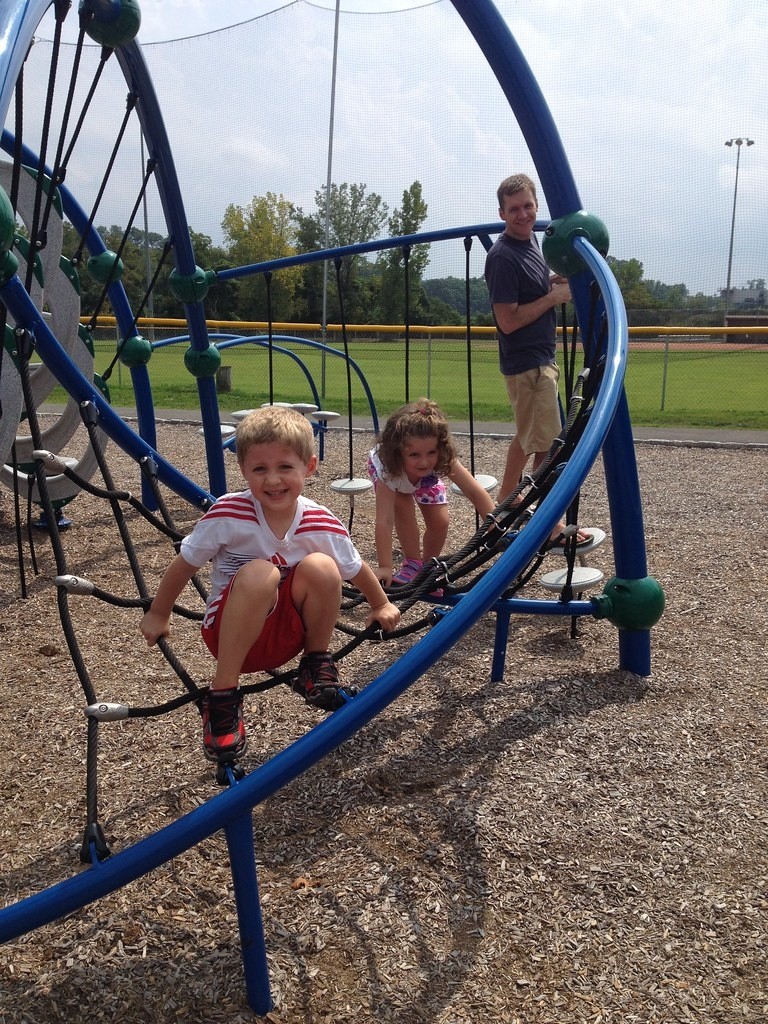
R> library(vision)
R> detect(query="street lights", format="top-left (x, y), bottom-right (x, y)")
top-left (724, 137), bottom-right (755, 315)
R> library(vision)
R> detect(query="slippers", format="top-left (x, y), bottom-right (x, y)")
top-left (494, 502), bottom-right (535, 516)
top-left (548, 535), bottom-right (594, 548)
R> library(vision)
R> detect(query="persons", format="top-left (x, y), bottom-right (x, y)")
top-left (483, 174), bottom-right (597, 550)
top-left (369, 397), bottom-right (500, 601)
top-left (137, 405), bottom-right (403, 763)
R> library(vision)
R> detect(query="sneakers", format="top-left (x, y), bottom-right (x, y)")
top-left (293, 651), bottom-right (358, 711)
top-left (203, 681), bottom-right (248, 762)
top-left (423, 561), bottom-right (443, 597)
top-left (389, 559), bottom-right (423, 587)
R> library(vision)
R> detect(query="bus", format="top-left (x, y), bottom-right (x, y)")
top-left (659, 335), bottom-right (710, 343)
